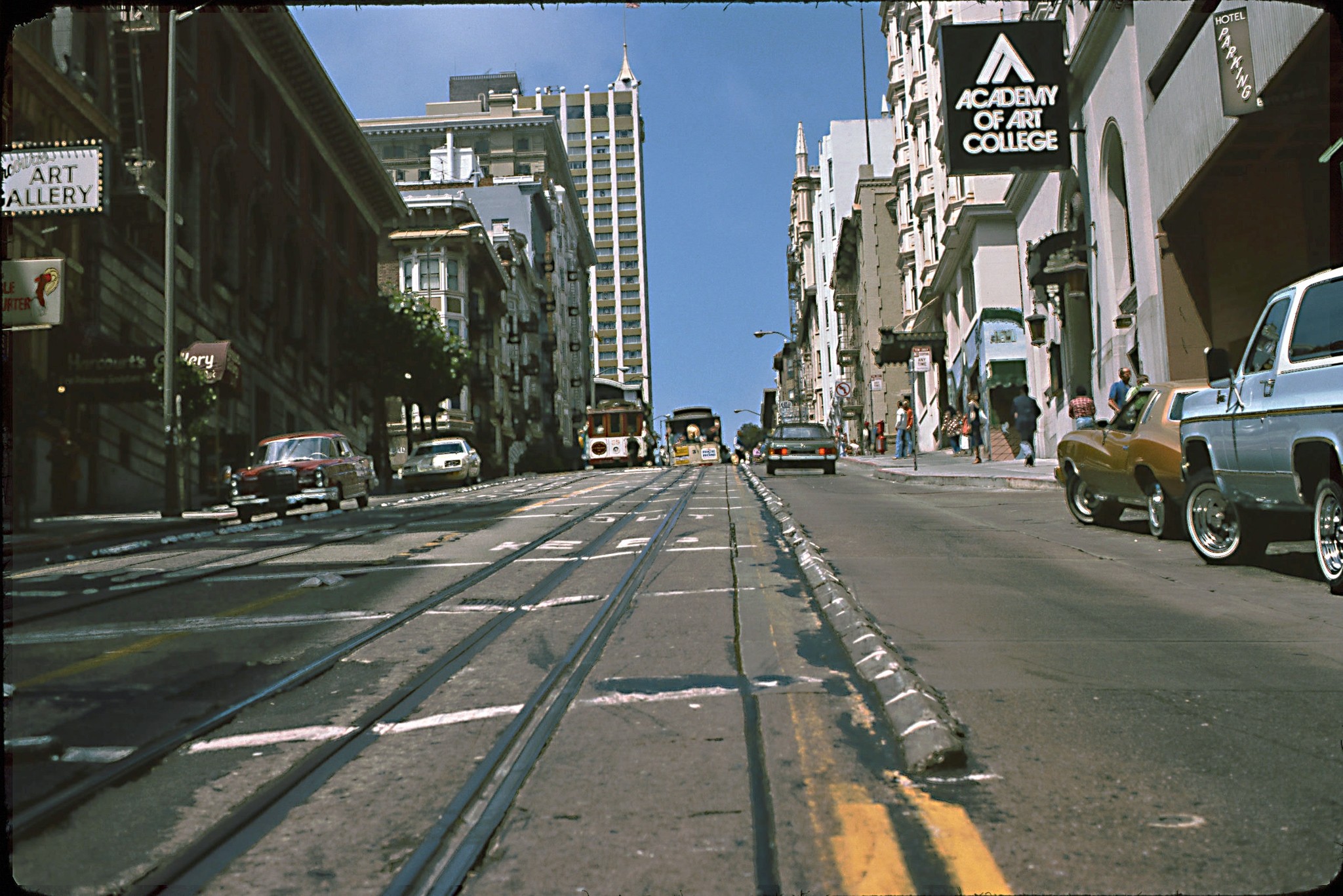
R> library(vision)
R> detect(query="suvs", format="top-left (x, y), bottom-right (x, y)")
top-left (1177, 264), bottom-right (1343, 595)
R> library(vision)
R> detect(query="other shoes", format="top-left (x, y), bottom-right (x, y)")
top-left (972, 457), bottom-right (981, 463)
top-left (1025, 453), bottom-right (1035, 467)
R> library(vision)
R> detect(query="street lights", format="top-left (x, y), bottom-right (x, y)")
top-left (752, 329), bottom-right (803, 424)
top-left (423, 219), bottom-right (483, 445)
top-left (590, 366), bottom-right (630, 410)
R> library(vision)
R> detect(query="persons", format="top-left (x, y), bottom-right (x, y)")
top-left (892, 400), bottom-right (913, 460)
top-left (1013, 384), bottom-right (1041, 467)
top-left (1069, 385), bottom-right (1095, 431)
top-left (733, 430), bottom-right (770, 465)
top-left (835, 421), bottom-right (870, 457)
top-left (627, 432), bottom-right (640, 467)
top-left (966, 392), bottom-right (984, 464)
top-left (1108, 368), bottom-right (1150, 414)
top-left (941, 405), bottom-right (966, 457)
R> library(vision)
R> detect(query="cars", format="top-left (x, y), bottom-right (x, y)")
top-left (1051, 379), bottom-right (1221, 542)
top-left (763, 422), bottom-right (839, 475)
top-left (228, 429), bottom-right (377, 524)
top-left (401, 437), bottom-right (483, 493)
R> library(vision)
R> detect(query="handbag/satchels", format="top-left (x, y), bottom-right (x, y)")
top-left (979, 409), bottom-right (987, 424)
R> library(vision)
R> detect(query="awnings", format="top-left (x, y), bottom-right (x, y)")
top-left (56, 342), bottom-right (243, 403)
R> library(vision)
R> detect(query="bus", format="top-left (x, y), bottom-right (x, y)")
top-left (665, 405), bottom-right (725, 466)
top-left (584, 397), bottom-right (659, 469)
